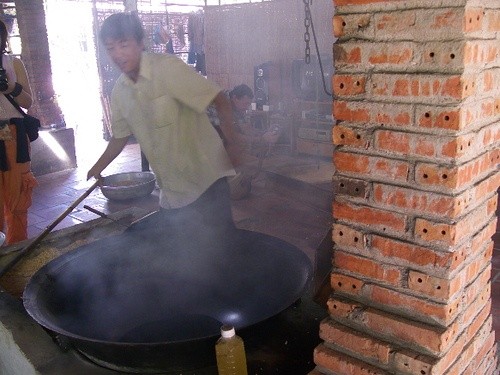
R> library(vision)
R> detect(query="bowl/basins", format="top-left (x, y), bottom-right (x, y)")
top-left (98, 171), bottom-right (156, 201)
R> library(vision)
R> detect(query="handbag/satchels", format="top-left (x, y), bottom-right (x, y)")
top-left (25, 115), bottom-right (41, 142)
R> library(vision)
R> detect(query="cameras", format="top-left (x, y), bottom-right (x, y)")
top-left (0, 66), bottom-right (8, 91)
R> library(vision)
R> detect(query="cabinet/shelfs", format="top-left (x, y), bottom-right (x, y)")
top-left (298, 98), bottom-right (335, 124)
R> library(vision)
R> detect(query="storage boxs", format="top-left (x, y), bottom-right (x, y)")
top-left (295, 128), bottom-right (334, 158)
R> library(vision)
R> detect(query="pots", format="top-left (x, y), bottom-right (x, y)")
top-left (27, 227), bottom-right (312, 368)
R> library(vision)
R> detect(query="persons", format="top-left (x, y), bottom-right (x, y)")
top-left (87, 12), bottom-right (284, 226)
top-left (0, 20), bottom-right (38, 248)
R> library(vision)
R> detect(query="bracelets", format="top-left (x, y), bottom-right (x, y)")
top-left (11, 83), bottom-right (23, 96)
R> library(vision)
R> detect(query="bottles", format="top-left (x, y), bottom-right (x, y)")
top-left (215, 324), bottom-right (248, 375)
